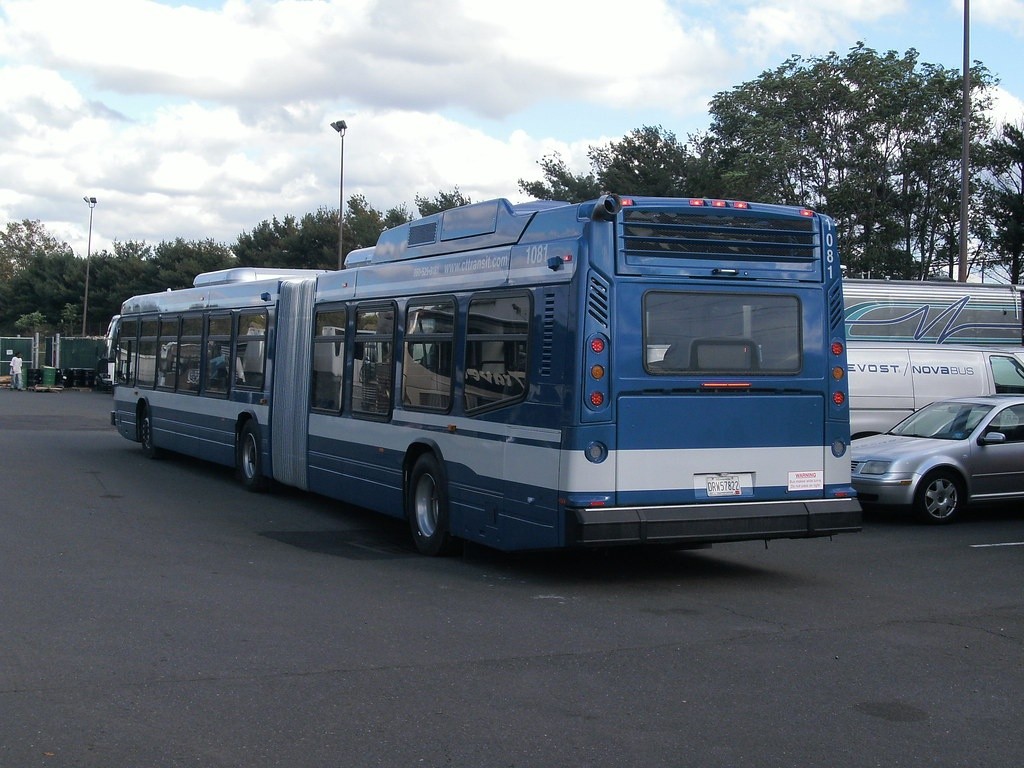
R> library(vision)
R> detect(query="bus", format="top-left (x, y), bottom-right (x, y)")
top-left (94, 192), bottom-right (865, 560)
top-left (741, 277), bottom-right (1024, 441)
top-left (376, 306), bottom-right (530, 412)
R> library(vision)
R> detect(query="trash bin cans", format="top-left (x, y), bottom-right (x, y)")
top-left (27, 368), bottom-right (43, 386)
top-left (14, 367), bottom-right (27, 387)
top-left (56, 368), bottom-right (94, 387)
top-left (40, 365), bottom-right (55, 385)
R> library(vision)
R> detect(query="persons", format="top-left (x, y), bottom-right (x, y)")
top-left (9, 352), bottom-right (23, 392)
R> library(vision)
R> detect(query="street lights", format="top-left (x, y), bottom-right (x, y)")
top-left (81, 195), bottom-right (98, 337)
top-left (329, 119), bottom-right (348, 271)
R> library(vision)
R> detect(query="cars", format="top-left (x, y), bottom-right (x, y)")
top-left (851, 393), bottom-right (1024, 526)
